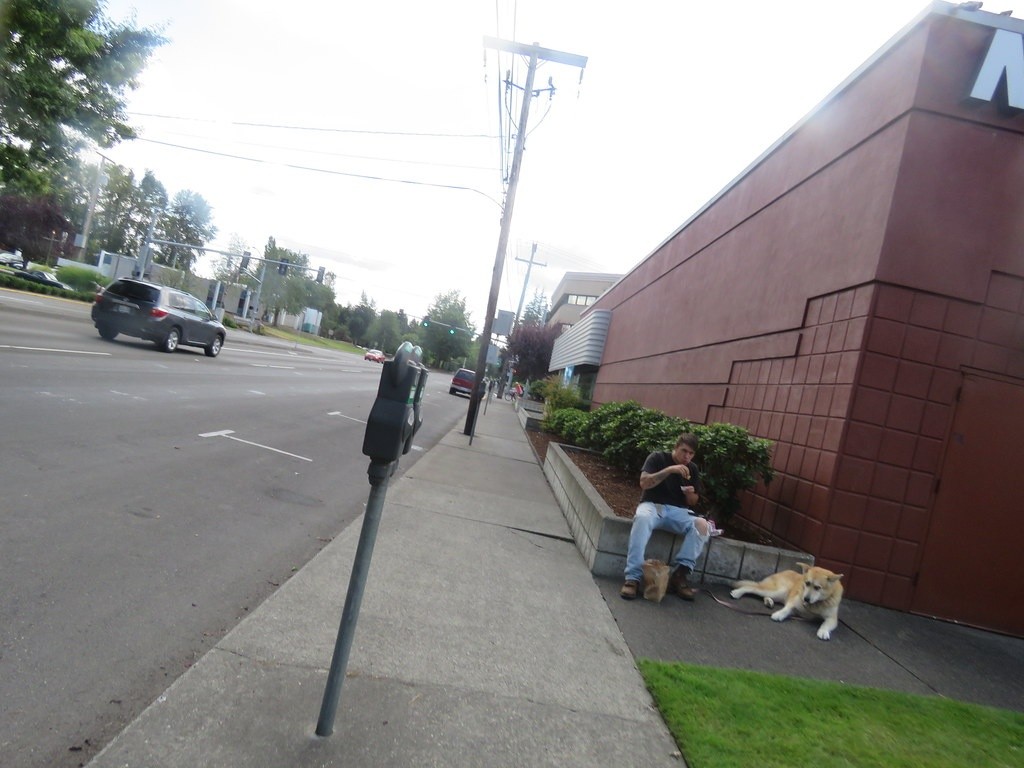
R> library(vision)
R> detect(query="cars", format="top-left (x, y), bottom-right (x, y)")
top-left (0, 253), bottom-right (23, 270)
top-left (13, 268), bottom-right (74, 290)
top-left (363, 348), bottom-right (385, 365)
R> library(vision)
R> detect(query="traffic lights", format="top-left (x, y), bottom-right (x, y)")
top-left (397, 309), bottom-right (403, 319)
top-left (422, 315), bottom-right (430, 327)
top-left (446, 318), bottom-right (457, 335)
top-left (240, 265), bottom-right (245, 275)
top-left (227, 255), bottom-right (234, 267)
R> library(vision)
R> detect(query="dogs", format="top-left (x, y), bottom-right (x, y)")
top-left (730, 562), bottom-right (845, 640)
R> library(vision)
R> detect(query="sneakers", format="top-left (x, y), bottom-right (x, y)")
top-left (620, 579), bottom-right (639, 598)
top-left (669, 574), bottom-right (695, 601)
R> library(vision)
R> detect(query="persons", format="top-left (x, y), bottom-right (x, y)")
top-left (620, 433), bottom-right (710, 601)
top-left (515, 382), bottom-right (525, 398)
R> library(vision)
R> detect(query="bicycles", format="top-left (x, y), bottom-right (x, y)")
top-left (504, 388), bottom-right (518, 401)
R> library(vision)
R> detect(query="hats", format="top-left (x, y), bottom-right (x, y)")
top-left (515, 382), bottom-right (518, 385)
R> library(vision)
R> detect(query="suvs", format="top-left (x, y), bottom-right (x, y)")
top-left (89, 276), bottom-right (227, 358)
top-left (449, 368), bottom-right (486, 400)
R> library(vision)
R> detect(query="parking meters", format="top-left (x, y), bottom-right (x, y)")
top-left (484, 379), bottom-right (494, 415)
top-left (309, 341), bottom-right (427, 738)
top-left (469, 381), bottom-right (487, 445)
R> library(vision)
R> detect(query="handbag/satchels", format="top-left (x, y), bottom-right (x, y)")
top-left (642, 558), bottom-right (670, 603)
